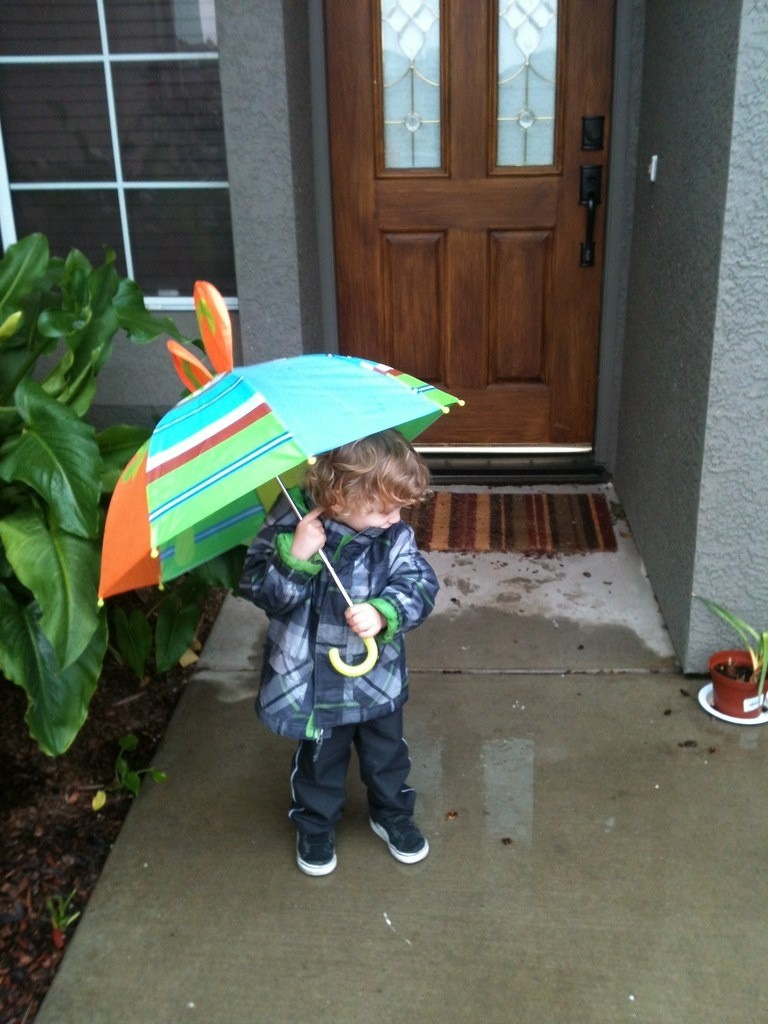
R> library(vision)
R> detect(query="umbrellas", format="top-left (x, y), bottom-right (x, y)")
top-left (97, 282), bottom-right (465, 678)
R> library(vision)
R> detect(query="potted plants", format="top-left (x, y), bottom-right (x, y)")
top-left (691, 595), bottom-right (768, 725)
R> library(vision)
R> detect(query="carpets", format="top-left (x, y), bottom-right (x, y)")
top-left (400, 493), bottom-right (618, 552)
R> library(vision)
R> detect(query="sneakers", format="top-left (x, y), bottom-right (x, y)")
top-left (295, 818), bottom-right (337, 878)
top-left (369, 812), bottom-right (429, 863)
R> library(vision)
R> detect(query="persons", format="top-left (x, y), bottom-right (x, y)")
top-left (239, 429), bottom-right (439, 876)
